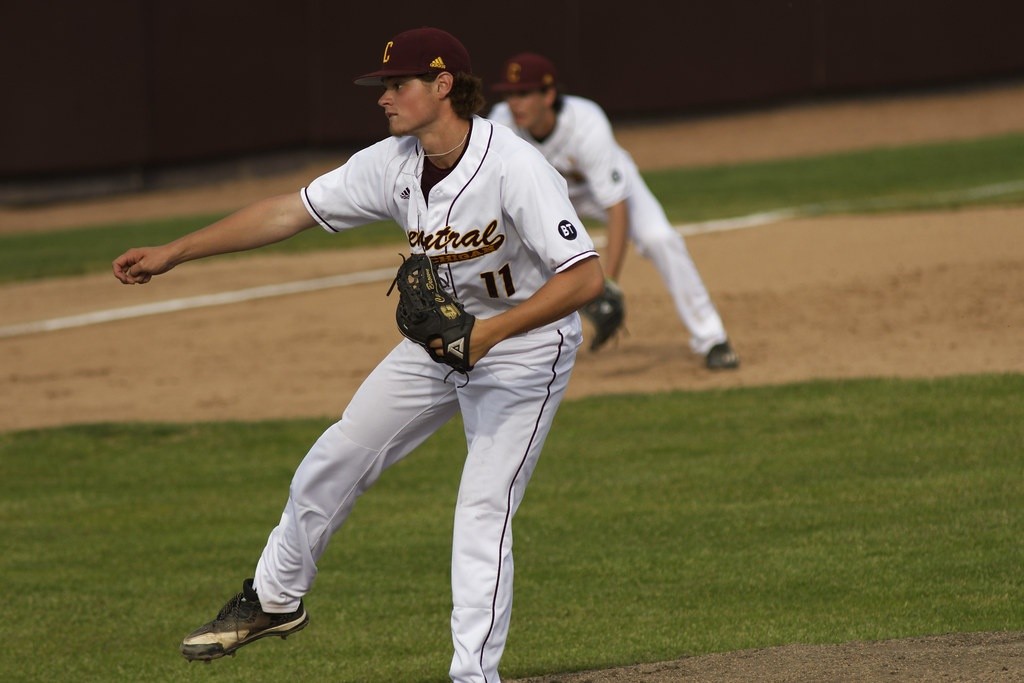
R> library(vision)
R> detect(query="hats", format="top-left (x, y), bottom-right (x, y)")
top-left (491, 54), bottom-right (554, 91)
top-left (351, 27), bottom-right (471, 86)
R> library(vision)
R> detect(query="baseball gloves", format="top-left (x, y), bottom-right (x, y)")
top-left (384, 251), bottom-right (476, 390)
top-left (581, 278), bottom-right (632, 353)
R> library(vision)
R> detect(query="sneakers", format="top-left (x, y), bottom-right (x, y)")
top-left (179, 578), bottom-right (309, 664)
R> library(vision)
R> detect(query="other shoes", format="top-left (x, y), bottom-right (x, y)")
top-left (705, 343), bottom-right (739, 368)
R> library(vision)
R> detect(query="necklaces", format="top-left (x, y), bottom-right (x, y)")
top-left (421, 128), bottom-right (470, 157)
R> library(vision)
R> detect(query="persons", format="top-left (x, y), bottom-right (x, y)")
top-left (111, 26), bottom-right (605, 683)
top-left (486, 52), bottom-right (740, 370)
